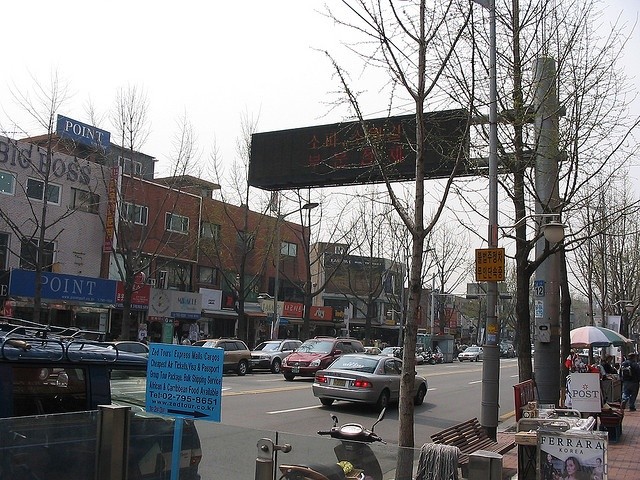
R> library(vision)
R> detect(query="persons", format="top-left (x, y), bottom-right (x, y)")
top-left (543, 454), bottom-right (558, 480)
top-left (590, 458), bottom-right (603, 480)
top-left (182, 333), bottom-right (191, 345)
top-left (561, 457), bottom-right (581, 480)
top-left (139, 337), bottom-right (148, 346)
top-left (601, 355), bottom-right (617, 374)
top-left (618, 352), bottom-right (640, 411)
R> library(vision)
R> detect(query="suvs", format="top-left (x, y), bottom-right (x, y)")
top-left (250, 337), bottom-right (304, 374)
top-left (281, 336), bottom-right (364, 380)
top-left (191, 337), bottom-right (251, 376)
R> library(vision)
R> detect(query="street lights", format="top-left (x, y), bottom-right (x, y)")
top-left (601, 300), bottom-right (634, 364)
top-left (386, 310), bottom-right (403, 346)
top-left (274, 203), bottom-right (319, 339)
top-left (481, 214), bottom-right (568, 443)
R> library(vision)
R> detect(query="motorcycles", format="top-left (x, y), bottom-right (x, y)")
top-left (421, 348), bottom-right (444, 365)
top-left (279, 407), bottom-right (387, 480)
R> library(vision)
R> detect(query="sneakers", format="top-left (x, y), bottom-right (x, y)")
top-left (621, 400), bottom-right (627, 409)
top-left (629, 407), bottom-right (636, 411)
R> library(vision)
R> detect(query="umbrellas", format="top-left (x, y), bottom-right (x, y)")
top-left (560, 325), bottom-right (628, 366)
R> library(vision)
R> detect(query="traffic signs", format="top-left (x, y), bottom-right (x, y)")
top-left (146, 342), bottom-right (224, 421)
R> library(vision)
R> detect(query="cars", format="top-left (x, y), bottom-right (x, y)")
top-left (378, 347), bottom-right (404, 357)
top-left (458, 345), bottom-right (483, 362)
top-left (312, 353), bottom-right (428, 412)
top-left (500, 343), bottom-right (515, 358)
top-left (107, 341), bottom-right (149, 371)
top-left (364, 347), bottom-right (382, 355)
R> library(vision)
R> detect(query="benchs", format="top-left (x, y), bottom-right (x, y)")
top-left (431, 417), bottom-right (517, 477)
